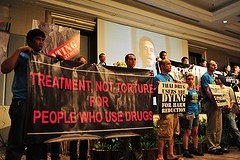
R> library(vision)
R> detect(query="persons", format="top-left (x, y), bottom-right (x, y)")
top-left (216, 76), bottom-right (240, 142)
top-left (37, 21), bottom-right (54, 54)
top-left (117, 53), bottom-right (154, 160)
top-left (1, 28), bottom-right (64, 160)
top-left (69, 56), bottom-right (88, 160)
top-left (154, 59), bottom-right (184, 160)
top-left (98, 53), bottom-right (107, 66)
top-left (180, 74), bottom-right (204, 158)
top-left (232, 65), bottom-right (240, 75)
top-left (138, 36), bottom-right (156, 70)
top-left (223, 65), bottom-right (230, 73)
top-left (155, 50), bottom-right (167, 112)
top-left (201, 59), bottom-right (229, 155)
top-left (182, 57), bottom-right (189, 64)
top-left (198, 59), bottom-right (207, 67)
top-left (49, 25), bottom-right (63, 49)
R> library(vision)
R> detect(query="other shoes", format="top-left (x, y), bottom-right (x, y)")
top-left (183, 152), bottom-right (193, 158)
top-left (168, 155), bottom-right (184, 160)
top-left (192, 148), bottom-right (204, 156)
top-left (218, 149), bottom-right (228, 152)
top-left (208, 150), bottom-right (223, 155)
